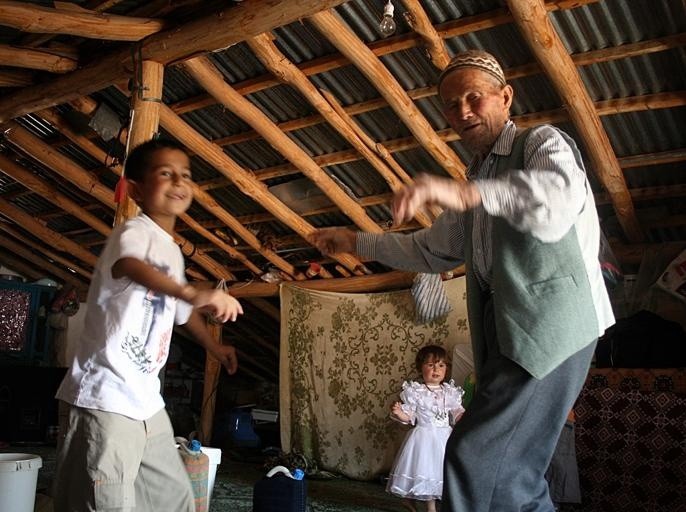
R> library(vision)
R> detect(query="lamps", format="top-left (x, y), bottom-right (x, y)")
top-left (379, 0), bottom-right (396, 36)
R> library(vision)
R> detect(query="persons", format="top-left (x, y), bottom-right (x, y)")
top-left (56, 138), bottom-right (244, 512)
top-left (305, 51), bottom-right (616, 511)
top-left (386, 346), bottom-right (465, 511)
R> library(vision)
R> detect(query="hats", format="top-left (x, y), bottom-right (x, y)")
top-left (437, 50), bottom-right (507, 85)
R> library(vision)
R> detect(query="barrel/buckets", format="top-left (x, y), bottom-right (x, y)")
top-left (0, 453), bottom-right (42, 512)
top-left (195, 447), bottom-right (221, 511)
top-left (178, 440), bottom-right (208, 512)
top-left (253, 465), bottom-right (306, 512)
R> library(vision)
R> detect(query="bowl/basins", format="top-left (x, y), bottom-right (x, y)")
top-left (0, 274), bottom-right (23, 283)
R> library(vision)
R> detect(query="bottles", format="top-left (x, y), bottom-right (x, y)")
top-left (624, 274), bottom-right (639, 304)
